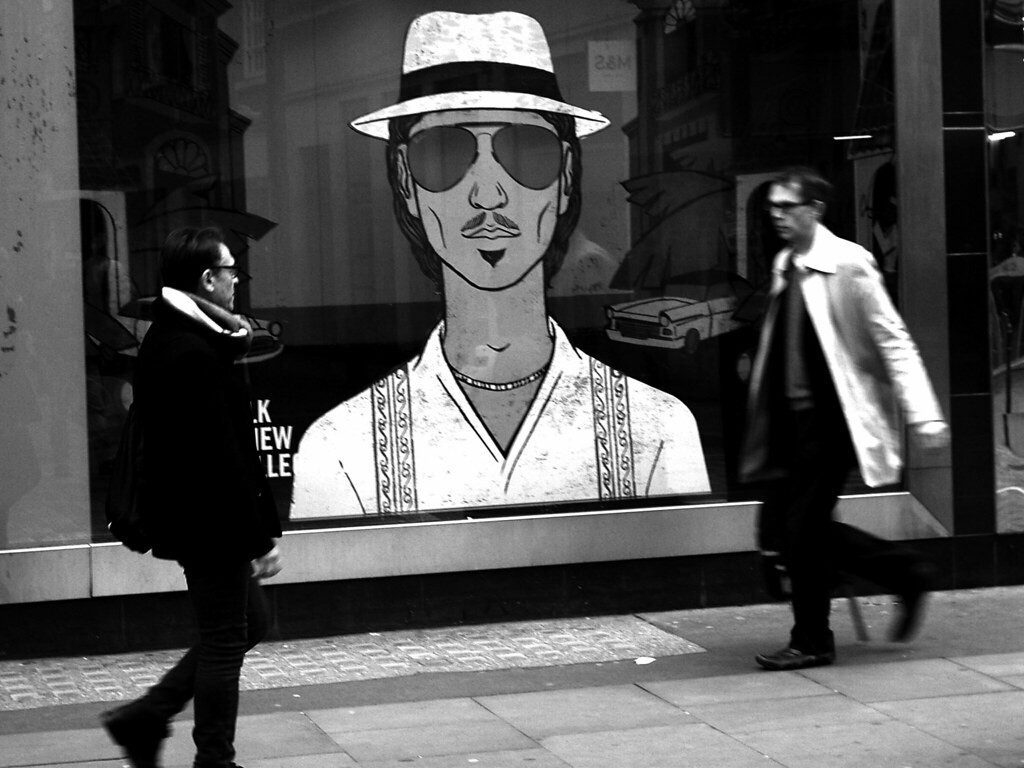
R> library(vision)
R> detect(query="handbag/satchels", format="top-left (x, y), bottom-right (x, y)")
top-left (107, 405), bottom-right (156, 552)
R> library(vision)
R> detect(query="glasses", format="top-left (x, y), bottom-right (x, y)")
top-left (768, 199), bottom-right (813, 211)
top-left (215, 261), bottom-right (246, 282)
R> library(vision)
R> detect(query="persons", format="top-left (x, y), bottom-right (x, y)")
top-left (98, 225), bottom-right (284, 768)
top-left (747, 172), bottom-right (951, 670)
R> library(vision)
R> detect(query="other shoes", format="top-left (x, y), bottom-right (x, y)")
top-left (100, 704), bottom-right (171, 766)
top-left (194, 755), bottom-right (240, 768)
top-left (754, 632), bottom-right (834, 669)
top-left (885, 567), bottom-right (924, 640)
